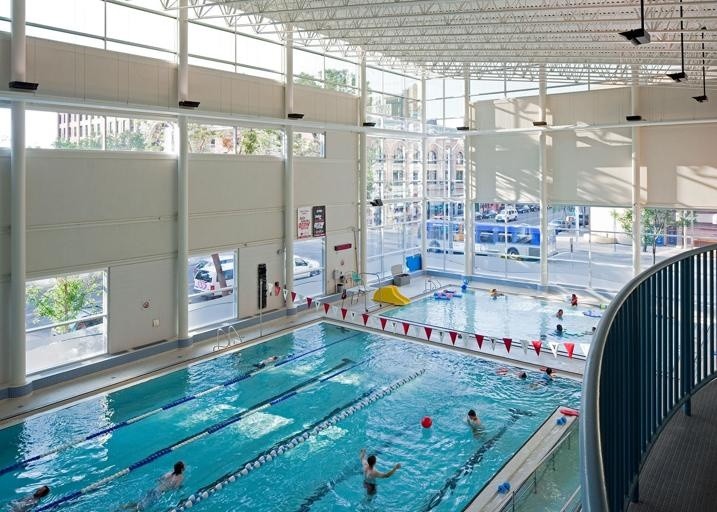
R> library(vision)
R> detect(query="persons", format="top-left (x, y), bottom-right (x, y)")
top-left (513, 371), bottom-right (527, 380)
top-left (250, 355), bottom-right (277, 371)
top-left (116, 460), bottom-right (185, 511)
top-left (464, 409), bottom-right (487, 442)
top-left (570, 293), bottom-right (578, 307)
top-left (547, 324), bottom-right (563, 337)
top-left (6, 484), bottom-right (50, 512)
top-left (549, 308), bottom-right (564, 321)
top-left (528, 367), bottom-right (552, 390)
top-left (488, 288), bottom-right (503, 296)
top-left (359, 448), bottom-right (401, 503)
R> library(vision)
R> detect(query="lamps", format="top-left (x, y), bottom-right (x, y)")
top-left (533, 121), bottom-right (548, 127)
top-left (626, 115), bottom-right (642, 121)
top-left (617, 1), bottom-right (710, 103)
top-left (8, 81), bottom-right (39, 91)
top-left (288, 112), bottom-right (305, 118)
top-left (362, 122), bottom-right (376, 128)
top-left (456, 126), bottom-right (470, 131)
top-left (179, 99), bottom-right (201, 108)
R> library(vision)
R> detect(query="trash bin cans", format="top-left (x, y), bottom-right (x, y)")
top-left (337, 284), bottom-right (343, 294)
top-left (394, 274), bottom-right (410, 287)
top-left (406, 255), bottom-right (421, 273)
top-left (668, 226), bottom-right (676, 244)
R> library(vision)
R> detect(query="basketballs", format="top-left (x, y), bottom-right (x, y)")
top-left (421, 416), bottom-right (433, 428)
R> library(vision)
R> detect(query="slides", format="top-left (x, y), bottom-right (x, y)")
top-left (390, 286), bottom-right (411, 305)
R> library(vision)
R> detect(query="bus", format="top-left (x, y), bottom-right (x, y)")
top-left (416, 218), bottom-right (558, 262)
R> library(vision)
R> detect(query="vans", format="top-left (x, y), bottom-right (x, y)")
top-left (496, 207), bottom-right (519, 221)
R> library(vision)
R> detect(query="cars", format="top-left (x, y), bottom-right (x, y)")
top-left (461, 209), bottom-right (497, 220)
top-left (192, 250), bottom-right (320, 296)
top-left (518, 205), bottom-right (540, 213)
top-left (549, 214), bottom-right (589, 228)
